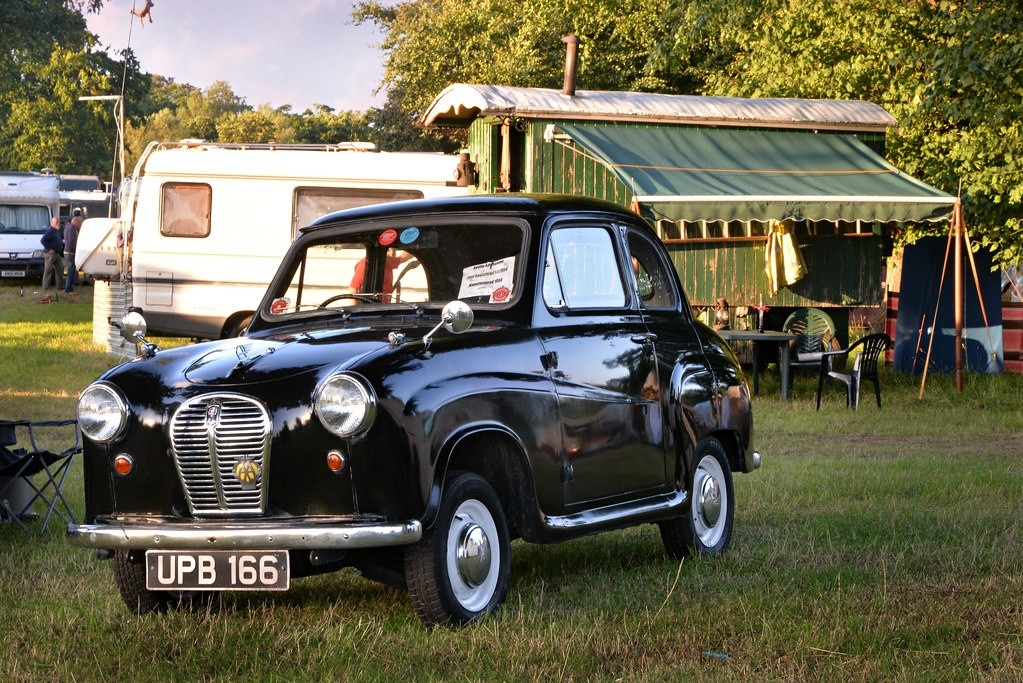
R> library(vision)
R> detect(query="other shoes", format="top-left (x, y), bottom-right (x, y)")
top-left (68, 292), bottom-right (79, 294)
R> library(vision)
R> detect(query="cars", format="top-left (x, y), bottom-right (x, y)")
top-left (65, 190), bottom-right (761, 628)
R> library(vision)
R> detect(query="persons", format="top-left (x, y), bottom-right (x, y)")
top-left (350, 247), bottom-right (414, 306)
top-left (41, 216), bottom-right (84, 295)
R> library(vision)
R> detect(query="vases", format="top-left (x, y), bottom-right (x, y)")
top-left (758, 315), bottom-right (764, 333)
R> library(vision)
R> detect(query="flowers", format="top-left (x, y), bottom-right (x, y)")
top-left (752, 302), bottom-right (770, 326)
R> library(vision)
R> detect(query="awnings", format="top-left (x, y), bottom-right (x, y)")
top-left (554, 122), bottom-right (961, 223)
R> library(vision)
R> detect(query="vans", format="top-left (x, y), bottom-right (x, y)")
top-left (0, 139), bottom-right (477, 341)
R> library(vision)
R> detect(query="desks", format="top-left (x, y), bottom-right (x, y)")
top-left (717, 331), bottom-right (797, 400)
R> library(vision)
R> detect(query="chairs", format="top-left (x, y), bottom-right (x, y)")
top-left (780, 308), bottom-right (836, 389)
top-left (0, 417), bottom-right (84, 536)
top-left (815, 332), bottom-right (891, 413)
